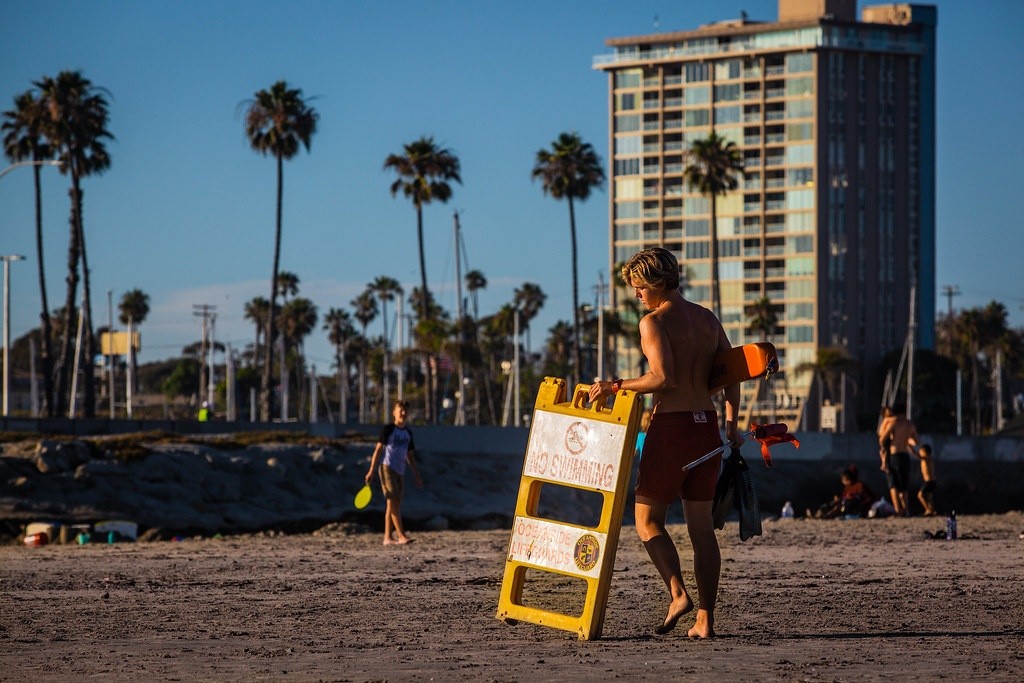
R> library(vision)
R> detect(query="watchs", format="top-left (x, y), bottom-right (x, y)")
top-left (612, 379), bottom-right (624, 392)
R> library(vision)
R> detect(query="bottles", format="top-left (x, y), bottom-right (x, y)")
top-left (946, 514), bottom-right (956, 540)
top-left (782, 501), bottom-right (794, 520)
top-left (23, 532), bottom-right (48, 546)
top-left (78, 531), bottom-right (114, 545)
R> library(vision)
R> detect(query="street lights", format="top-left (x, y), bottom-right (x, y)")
top-left (0, 253), bottom-right (27, 418)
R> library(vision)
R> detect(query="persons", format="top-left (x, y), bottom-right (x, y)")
top-left (589, 247), bottom-right (746, 638)
top-left (878, 400), bottom-right (919, 519)
top-left (198, 400), bottom-right (216, 422)
top-left (633, 409), bottom-right (652, 459)
top-left (834, 464), bottom-right (871, 519)
top-left (365, 400), bottom-right (423, 544)
top-left (907, 442), bottom-right (938, 516)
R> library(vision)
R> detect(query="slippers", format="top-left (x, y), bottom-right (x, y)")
top-left (912, 529), bottom-right (947, 539)
